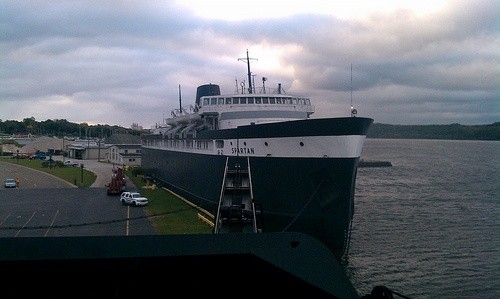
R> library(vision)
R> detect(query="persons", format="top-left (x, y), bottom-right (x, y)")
top-left (15, 177), bottom-right (20, 187)
top-left (74, 176), bottom-right (77, 185)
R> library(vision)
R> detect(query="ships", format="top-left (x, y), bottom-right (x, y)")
top-left (140, 48), bottom-right (374, 266)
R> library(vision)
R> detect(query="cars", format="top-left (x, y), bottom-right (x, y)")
top-left (4, 179), bottom-right (17, 188)
top-left (13, 154), bottom-right (47, 160)
top-left (64, 160), bottom-right (78, 167)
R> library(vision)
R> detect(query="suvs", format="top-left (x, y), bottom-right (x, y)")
top-left (119, 192), bottom-right (149, 207)
